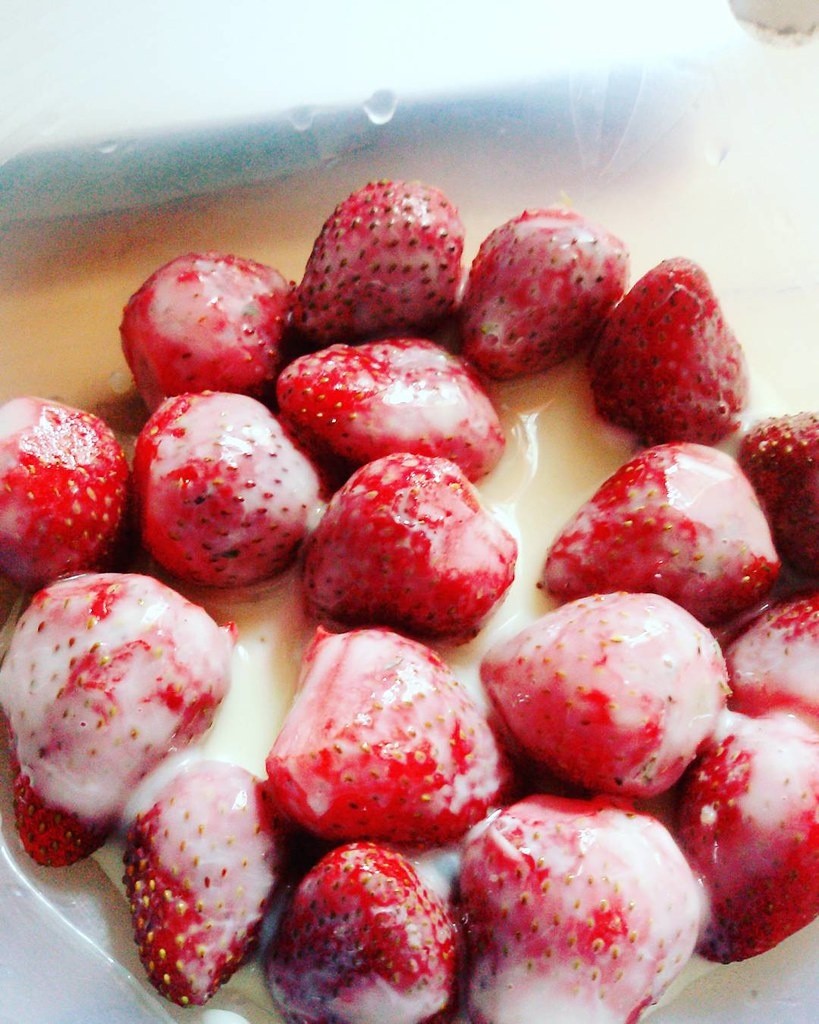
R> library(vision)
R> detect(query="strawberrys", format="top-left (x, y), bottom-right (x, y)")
top-left (0, 179), bottom-right (819, 1023)
